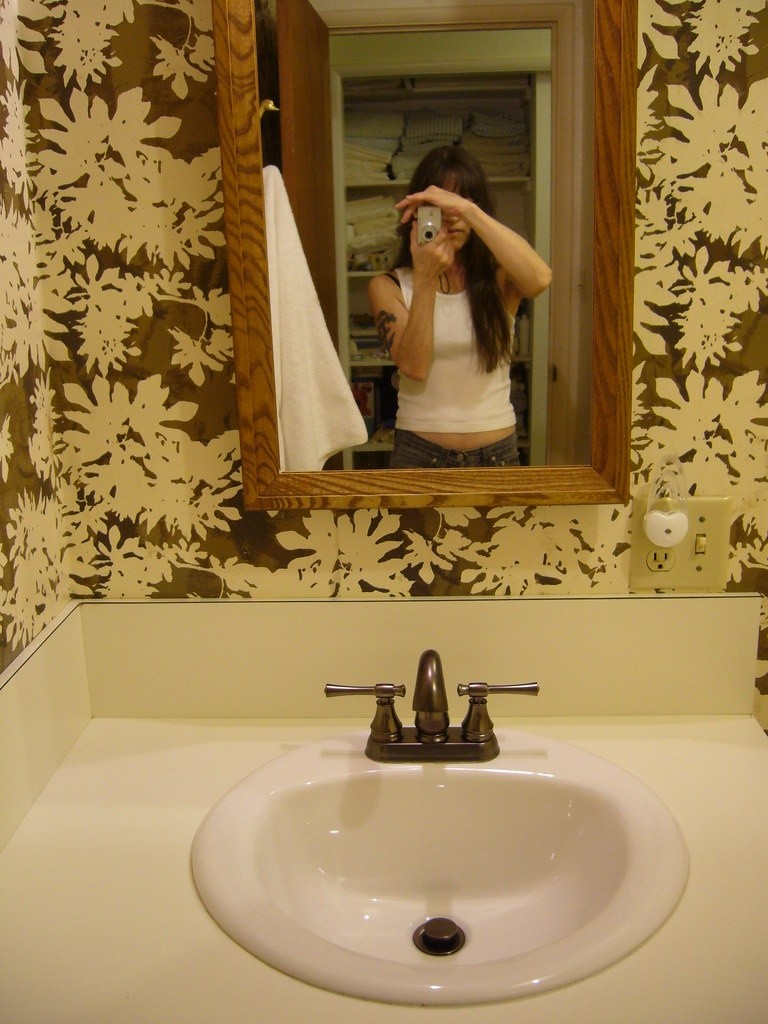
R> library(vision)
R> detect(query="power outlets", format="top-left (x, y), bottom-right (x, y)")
top-left (627, 496), bottom-right (731, 590)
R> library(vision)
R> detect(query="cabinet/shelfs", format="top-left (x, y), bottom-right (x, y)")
top-left (340, 82), bottom-right (530, 455)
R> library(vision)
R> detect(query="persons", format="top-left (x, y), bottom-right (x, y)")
top-left (368, 144), bottom-right (554, 468)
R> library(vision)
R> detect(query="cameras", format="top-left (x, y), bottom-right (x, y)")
top-left (417, 206), bottom-right (441, 245)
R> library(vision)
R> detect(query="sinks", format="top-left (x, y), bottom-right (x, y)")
top-left (189, 724), bottom-right (694, 1005)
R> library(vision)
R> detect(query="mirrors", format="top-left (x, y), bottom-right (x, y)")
top-left (210, 0), bottom-right (636, 509)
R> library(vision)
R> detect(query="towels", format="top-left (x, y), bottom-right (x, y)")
top-left (339, 101), bottom-right (538, 366)
top-left (263, 163), bottom-right (369, 471)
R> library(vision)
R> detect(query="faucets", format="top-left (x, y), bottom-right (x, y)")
top-left (412, 649), bottom-right (451, 744)
